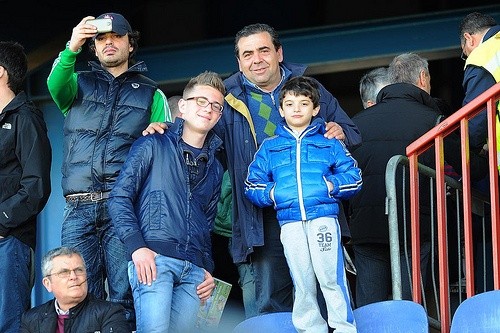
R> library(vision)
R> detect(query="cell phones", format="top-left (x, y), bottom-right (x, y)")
top-left (86, 18), bottom-right (113, 33)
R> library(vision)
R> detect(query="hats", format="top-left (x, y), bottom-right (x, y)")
top-left (92, 13), bottom-right (132, 41)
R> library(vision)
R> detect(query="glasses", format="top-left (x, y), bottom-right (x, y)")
top-left (185, 97), bottom-right (224, 113)
top-left (461, 32), bottom-right (474, 61)
top-left (46, 266), bottom-right (86, 278)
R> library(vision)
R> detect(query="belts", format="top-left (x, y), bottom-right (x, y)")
top-left (66, 192), bottom-right (111, 202)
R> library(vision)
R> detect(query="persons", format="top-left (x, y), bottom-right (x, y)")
top-left (0, 41), bottom-right (53, 333)
top-left (243, 78), bottom-right (362, 332)
top-left (46, 13), bottom-right (172, 333)
top-left (461, 9), bottom-right (500, 292)
top-left (142, 23), bottom-right (362, 319)
top-left (22, 246), bottom-right (130, 333)
top-left (359, 67), bottom-right (391, 110)
top-left (351, 52), bottom-right (462, 309)
top-left (167, 96), bottom-right (184, 123)
top-left (212, 170), bottom-right (257, 320)
top-left (109, 71), bottom-right (223, 332)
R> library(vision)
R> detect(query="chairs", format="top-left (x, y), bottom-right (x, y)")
top-left (353, 300), bottom-right (430, 333)
top-left (451, 290), bottom-right (500, 333)
